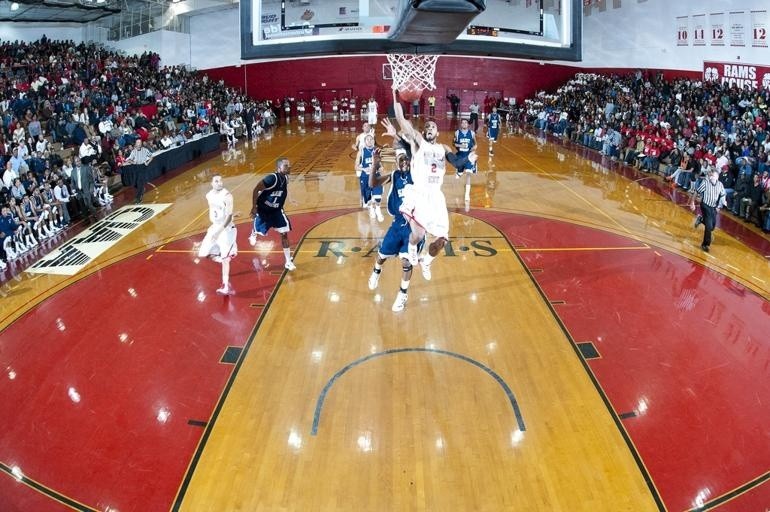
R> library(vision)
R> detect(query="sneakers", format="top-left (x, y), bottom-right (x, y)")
top-left (368, 266), bottom-right (382, 289)
top-left (7, 227), bottom-right (60, 259)
top-left (392, 292), bottom-right (408, 312)
top-left (369, 209), bottom-right (384, 222)
top-left (98, 193), bottom-right (113, 205)
top-left (408, 243), bottom-right (431, 281)
top-left (249, 234), bottom-right (257, 246)
top-left (285, 262), bottom-right (296, 270)
top-left (216, 287), bottom-right (228, 295)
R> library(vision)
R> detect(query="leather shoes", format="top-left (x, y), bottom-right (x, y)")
top-left (695, 216), bottom-right (702, 228)
top-left (701, 244), bottom-right (708, 251)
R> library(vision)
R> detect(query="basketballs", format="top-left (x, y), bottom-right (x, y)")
top-left (399, 78), bottom-right (426, 102)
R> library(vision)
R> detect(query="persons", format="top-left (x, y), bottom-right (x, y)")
top-left (200, 73), bottom-right (380, 148)
top-left (368, 116), bottom-right (414, 314)
top-left (353, 134), bottom-right (386, 223)
top-left (688, 168), bottom-right (727, 253)
top-left (413, 92), bottom-right (527, 201)
top-left (248, 155), bottom-right (299, 271)
top-left (528, 67), bottom-right (770, 234)
top-left (0, 34), bottom-right (200, 272)
top-left (671, 253), bottom-right (708, 321)
top-left (392, 84), bottom-right (481, 281)
top-left (198, 174), bottom-right (241, 296)
